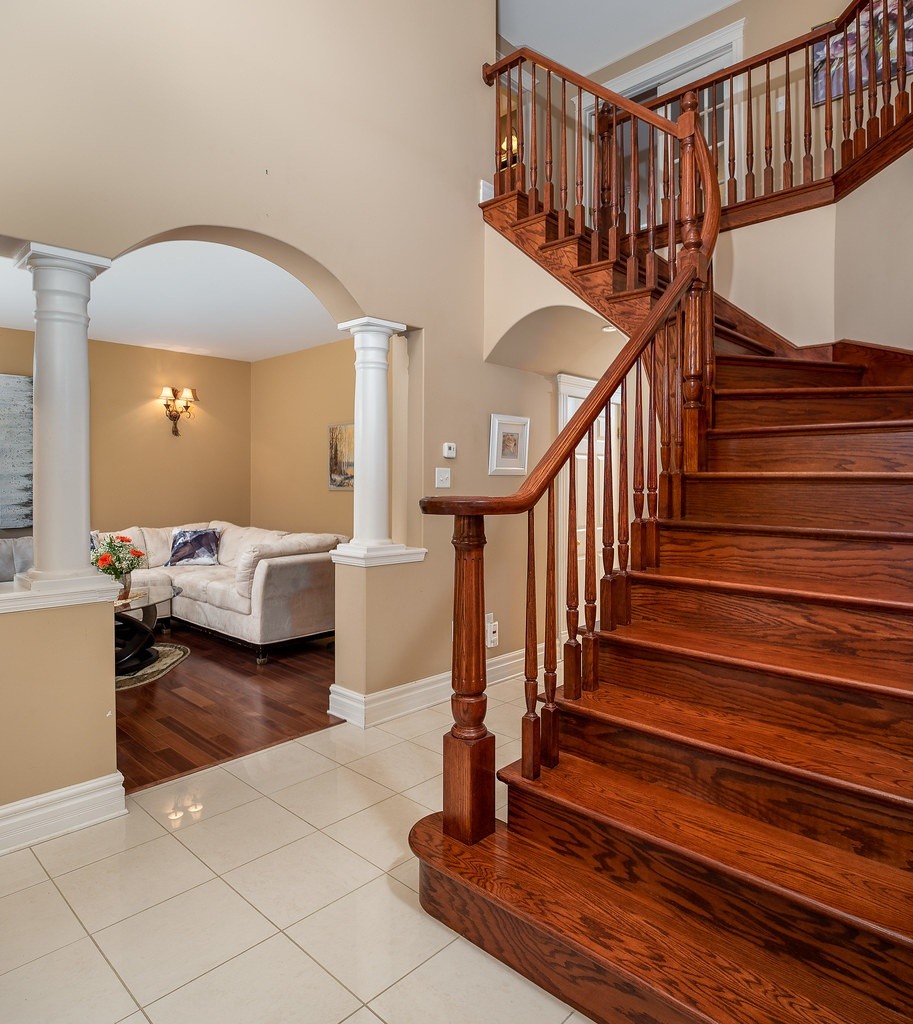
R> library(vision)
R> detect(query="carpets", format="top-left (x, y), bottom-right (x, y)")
top-left (114, 642), bottom-right (191, 690)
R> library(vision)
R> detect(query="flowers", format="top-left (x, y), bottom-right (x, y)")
top-left (92, 535), bottom-right (147, 577)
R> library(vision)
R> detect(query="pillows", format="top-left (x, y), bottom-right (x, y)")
top-left (162, 526), bottom-right (226, 566)
top-left (90, 529), bottom-right (100, 555)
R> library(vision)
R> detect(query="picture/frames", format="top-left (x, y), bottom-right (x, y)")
top-left (488, 413), bottom-right (530, 476)
top-left (326, 421), bottom-right (355, 491)
top-left (810, 0), bottom-right (913, 109)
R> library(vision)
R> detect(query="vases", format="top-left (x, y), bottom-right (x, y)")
top-left (115, 572), bottom-right (132, 599)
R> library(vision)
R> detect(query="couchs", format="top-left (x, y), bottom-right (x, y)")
top-left (0, 519), bottom-right (352, 665)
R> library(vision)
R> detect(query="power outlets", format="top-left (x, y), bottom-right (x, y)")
top-left (486, 613), bottom-right (494, 631)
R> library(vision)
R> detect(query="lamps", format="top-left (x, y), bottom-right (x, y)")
top-left (158, 386), bottom-right (195, 436)
top-left (501, 126), bottom-right (517, 151)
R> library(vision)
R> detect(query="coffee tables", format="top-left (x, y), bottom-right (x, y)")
top-left (115, 586), bottom-right (184, 674)
